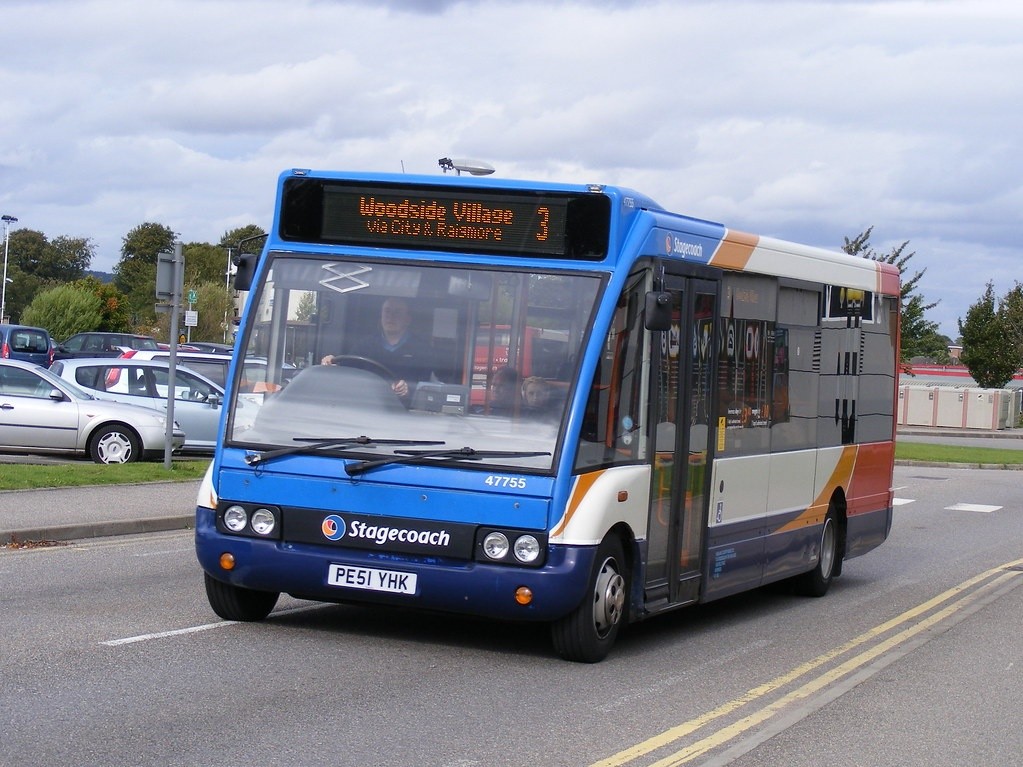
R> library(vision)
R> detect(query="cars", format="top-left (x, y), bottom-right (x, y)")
top-left (35, 358), bottom-right (263, 452)
top-left (0, 358), bottom-right (185, 464)
top-left (155, 341), bottom-right (254, 361)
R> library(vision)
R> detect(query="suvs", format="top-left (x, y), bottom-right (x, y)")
top-left (51, 332), bottom-right (158, 382)
top-left (107, 346), bottom-right (287, 407)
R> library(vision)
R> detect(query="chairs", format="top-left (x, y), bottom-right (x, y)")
top-left (134, 375), bottom-right (147, 395)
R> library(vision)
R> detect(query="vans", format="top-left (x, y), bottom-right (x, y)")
top-left (0, 324), bottom-right (52, 386)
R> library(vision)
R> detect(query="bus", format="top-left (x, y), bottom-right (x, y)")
top-left (197, 167), bottom-right (903, 664)
top-left (462, 325), bottom-right (569, 415)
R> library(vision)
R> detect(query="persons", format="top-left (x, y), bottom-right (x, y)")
top-left (520, 375), bottom-right (565, 420)
top-left (320, 296), bottom-right (439, 398)
top-left (178, 334), bottom-right (187, 344)
top-left (468, 366), bottom-right (517, 423)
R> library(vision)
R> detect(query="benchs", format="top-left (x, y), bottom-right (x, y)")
top-left (521, 378), bottom-right (611, 443)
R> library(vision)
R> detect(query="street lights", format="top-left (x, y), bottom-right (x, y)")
top-left (1, 215), bottom-right (18, 323)
top-left (219, 244), bottom-right (239, 343)
